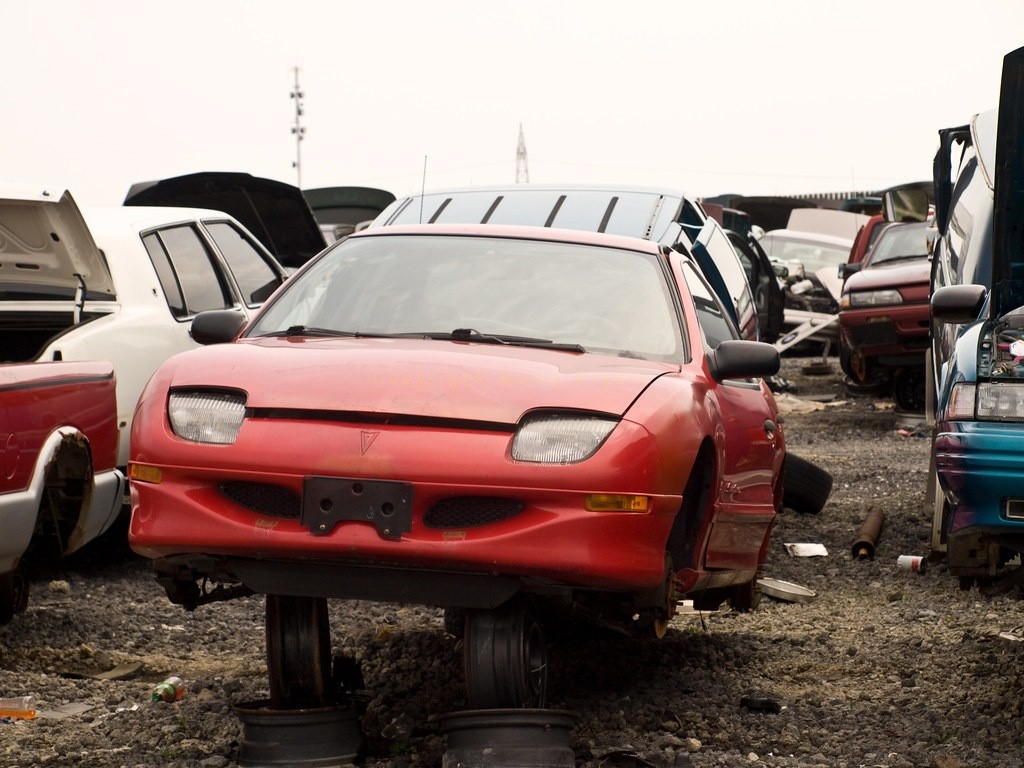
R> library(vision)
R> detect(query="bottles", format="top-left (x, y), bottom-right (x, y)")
top-left (0, 696), bottom-right (36, 719)
top-left (152, 677), bottom-right (185, 702)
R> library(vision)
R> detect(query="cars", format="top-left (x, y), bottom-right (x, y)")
top-left (123, 169), bottom-right (929, 407)
top-left (130, 224), bottom-right (789, 705)
top-left (0, 186), bottom-right (296, 575)
top-left (926, 48), bottom-right (1024, 589)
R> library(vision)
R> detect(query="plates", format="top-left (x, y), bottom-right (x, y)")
top-left (756, 577), bottom-right (816, 603)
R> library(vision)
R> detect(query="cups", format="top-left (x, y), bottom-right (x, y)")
top-left (791, 279), bottom-right (813, 295)
top-left (897, 555), bottom-right (927, 575)
